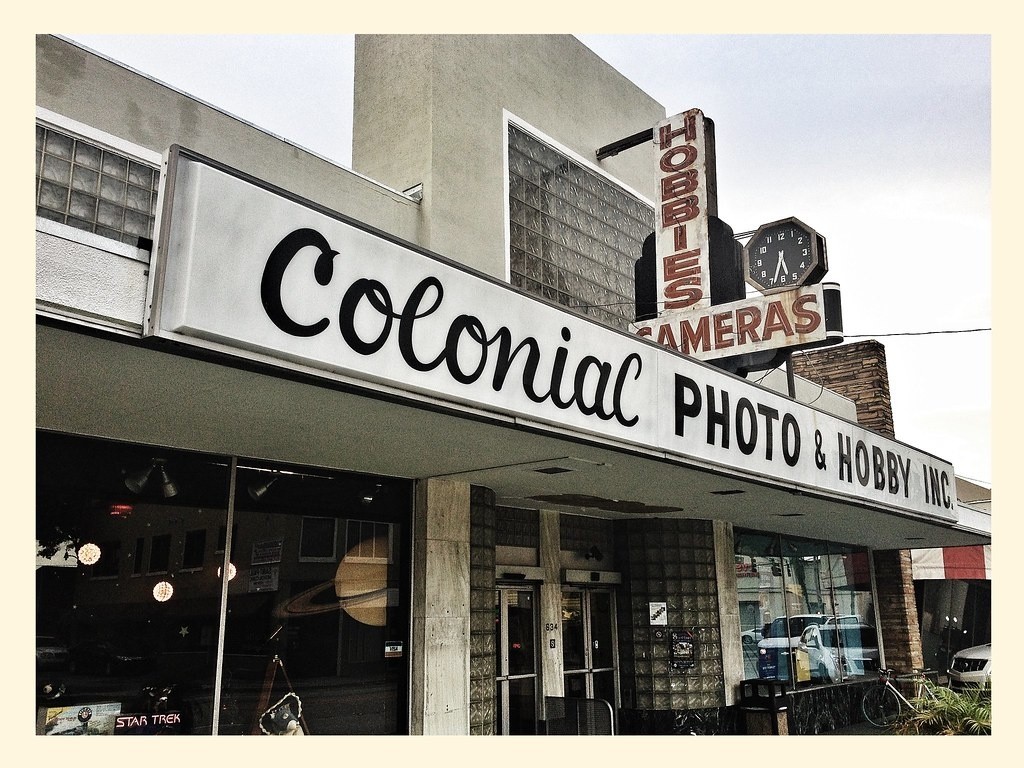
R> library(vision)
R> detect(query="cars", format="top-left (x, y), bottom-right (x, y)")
top-left (740, 614), bottom-right (881, 684)
top-left (70, 642), bottom-right (147, 679)
top-left (36, 634), bottom-right (73, 675)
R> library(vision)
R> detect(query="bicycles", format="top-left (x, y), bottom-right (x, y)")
top-left (862, 668), bottom-right (940, 727)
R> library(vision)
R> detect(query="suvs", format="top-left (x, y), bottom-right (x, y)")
top-left (943, 642), bottom-right (991, 694)
